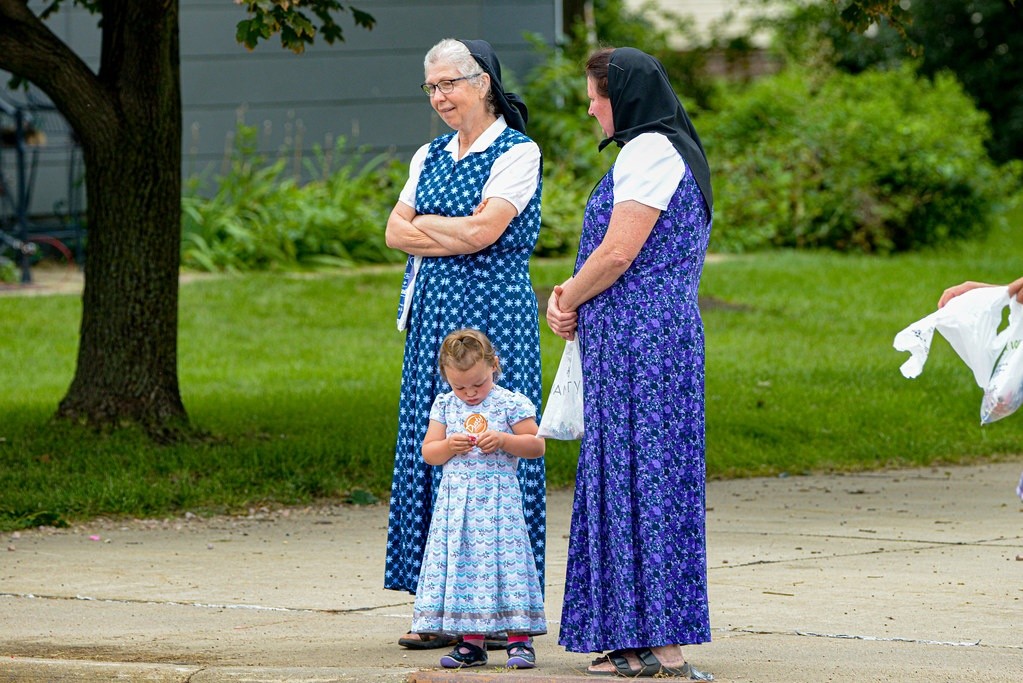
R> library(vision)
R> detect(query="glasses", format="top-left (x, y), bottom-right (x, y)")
top-left (420, 73), bottom-right (482, 96)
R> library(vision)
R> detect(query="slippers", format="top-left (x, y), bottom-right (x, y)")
top-left (399, 629), bottom-right (455, 650)
top-left (587, 646), bottom-right (691, 678)
top-left (485, 635), bottom-right (535, 649)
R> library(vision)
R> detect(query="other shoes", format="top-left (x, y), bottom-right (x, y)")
top-left (440, 642), bottom-right (488, 668)
top-left (506, 641), bottom-right (536, 667)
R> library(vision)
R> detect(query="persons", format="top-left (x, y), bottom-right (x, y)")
top-left (544, 45), bottom-right (712, 677)
top-left (382, 35), bottom-right (545, 647)
top-left (411, 330), bottom-right (545, 669)
top-left (937, 276), bottom-right (1023, 306)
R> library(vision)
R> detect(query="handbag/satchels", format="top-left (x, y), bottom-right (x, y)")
top-left (979, 297), bottom-right (1023, 425)
top-left (535, 332), bottom-right (586, 441)
